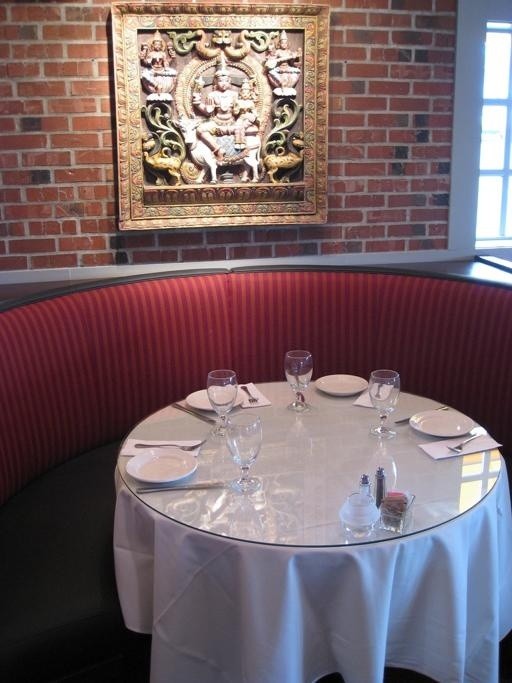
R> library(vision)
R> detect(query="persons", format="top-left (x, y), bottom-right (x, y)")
top-left (267, 35), bottom-right (303, 89)
top-left (139, 34), bottom-right (178, 94)
top-left (231, 79), bottom-right (257, 150)
top-left (193, 62), bottom-right (258, 160)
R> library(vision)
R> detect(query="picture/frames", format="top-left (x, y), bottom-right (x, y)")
top-left (105, 1), bottom-right (330, 230)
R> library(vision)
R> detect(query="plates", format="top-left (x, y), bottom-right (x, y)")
top-left (187, 389), bottom-right (244, 411)
top-left (409, 411), bottom-right (475, 437)
top-left (340, 503), bottom-right (381, 526)
top-left (315, 375), bottom-right (368, 395)
top-left (128, 449), bottom-right (198, 482)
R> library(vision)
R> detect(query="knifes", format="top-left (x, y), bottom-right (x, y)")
top-left (394, 406), bottom-right (449, 424)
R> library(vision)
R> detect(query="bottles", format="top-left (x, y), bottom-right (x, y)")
top-left (376, 468), bottom-right (386, 503)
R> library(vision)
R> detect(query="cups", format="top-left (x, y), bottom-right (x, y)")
top-left (348, 494), bottom-right (376, 516)
top-left (377, 490), bottom-right (416, 536)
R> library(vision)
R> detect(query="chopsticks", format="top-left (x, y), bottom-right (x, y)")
top-left (172, 404), bottom-right (215, 426)
top-left (137, 483), bottom-right (226, 494)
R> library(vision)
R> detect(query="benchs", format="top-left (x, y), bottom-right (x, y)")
top-left (2, 265), bottom-right (510, 683)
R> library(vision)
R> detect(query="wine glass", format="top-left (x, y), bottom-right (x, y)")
top-left (224, 413), bottom-right (263, 491)
top-left (208, 349), bottom-right (401, 493)
top-left (284, 351), bottom-right (313, 413)
top-left (369, 370), bottom-right (398, 439)
top-left (208, 370), bottom-right (237, 438)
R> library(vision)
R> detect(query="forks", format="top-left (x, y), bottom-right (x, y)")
top-left (134, 440), bottom-right (206, 451)
top-left (240, 385), bottom-right (259, 404)
top-left (446, 434), bottom-right (475, 453)
